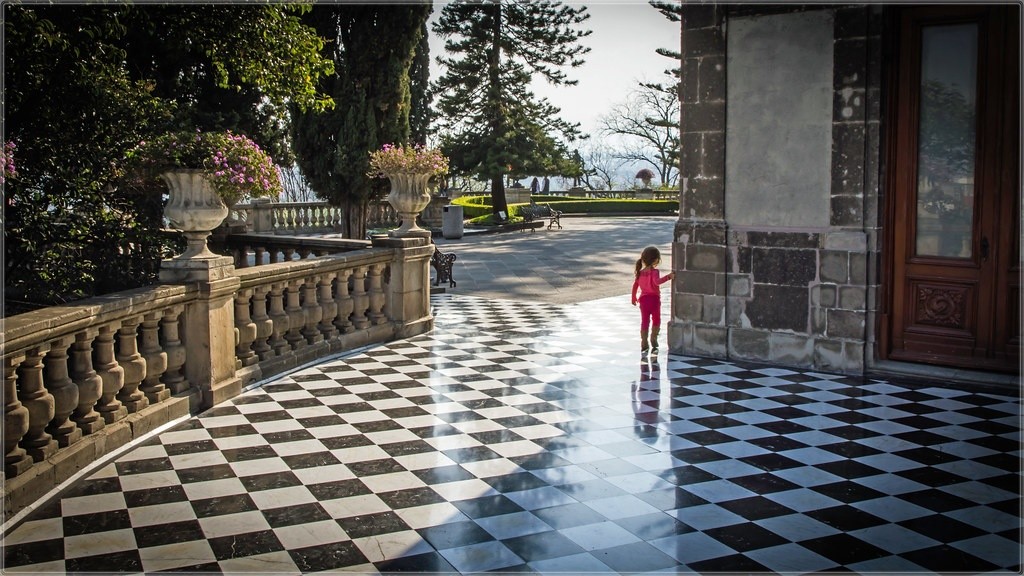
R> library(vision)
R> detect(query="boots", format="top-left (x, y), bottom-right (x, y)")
top-left (641, 332), bottom-right (649, 351)
top-left (651, 326), bottom-right (660, 347)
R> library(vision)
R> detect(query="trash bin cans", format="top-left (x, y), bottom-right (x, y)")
top-left (441, 205), bottom-right (464, 240)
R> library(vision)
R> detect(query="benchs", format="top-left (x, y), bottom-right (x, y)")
top-left (362, 234), bottom-right (457, 288)
top-left (517, 204), bottom-right (562, 232)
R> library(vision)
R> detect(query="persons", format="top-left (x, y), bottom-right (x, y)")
top-left (631, 247), bottom-right (675, 349)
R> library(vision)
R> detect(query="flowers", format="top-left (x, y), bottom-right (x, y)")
top-left (124, 129), bottom-right (283, 198)
top-left (365, 143), bottom-right (450, 177)
